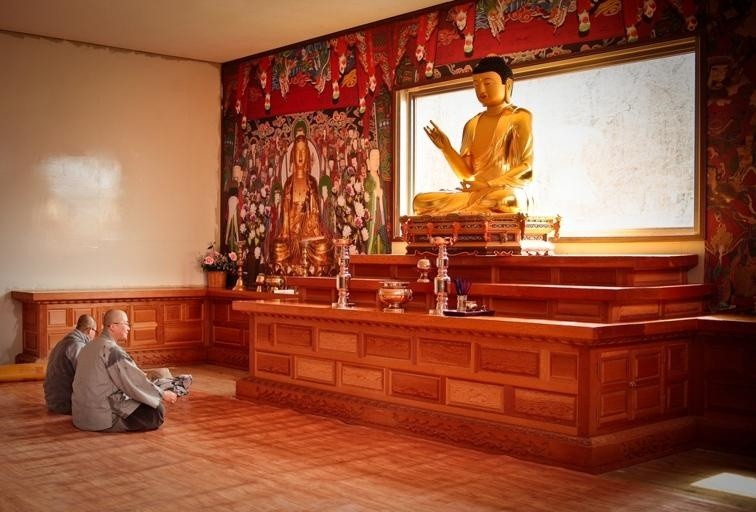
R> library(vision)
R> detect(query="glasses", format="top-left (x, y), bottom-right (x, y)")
top-left (108, 323), bottom-right (131, 327)
top-left (84, 328), bottom-right (99, 335)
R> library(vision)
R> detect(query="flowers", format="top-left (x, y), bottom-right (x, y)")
top-left (197, 241), bottom-right (238, 276)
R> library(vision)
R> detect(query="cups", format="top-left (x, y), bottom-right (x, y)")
top-left (463, 301), bottom-right (479, 312)
top-left (457, 295), bottom-right (467, 311)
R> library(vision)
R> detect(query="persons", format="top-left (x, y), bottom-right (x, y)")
top-left (42, 314), bottom-right (97, 415)
top-left (223, 118), bottom-right (390, 274)
top-left (69, 308), bottom-right (178, 434)
top-left (410, 56), bottom-right (534, 215)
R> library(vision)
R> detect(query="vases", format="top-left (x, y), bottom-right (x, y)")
top-left (207, 270), bottom-right (227, 288)
